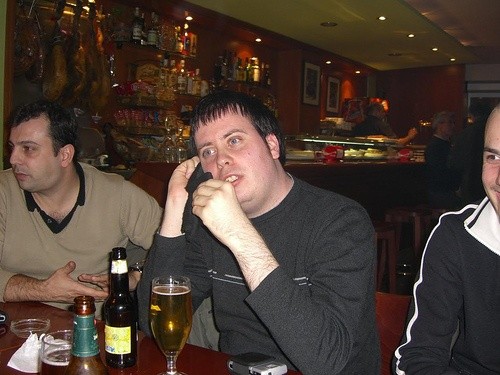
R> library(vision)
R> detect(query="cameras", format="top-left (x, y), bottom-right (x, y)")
top-left (227, 353), bottom-right (287, 375)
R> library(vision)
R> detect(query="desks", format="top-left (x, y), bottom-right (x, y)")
top-left (0, 301), bottom-right (305, 375)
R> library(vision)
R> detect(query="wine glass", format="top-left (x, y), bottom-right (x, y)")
top-left (151, 275), bottom-right (193, 375)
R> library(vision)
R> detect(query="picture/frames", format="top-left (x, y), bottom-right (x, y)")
top-left (302, 61), bottom-right (322, 106)
top-left (325, 75), bottom-right (342, 114)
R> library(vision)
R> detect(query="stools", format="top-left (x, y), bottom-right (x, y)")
top-left (373, 204), bottom-right (450, 295)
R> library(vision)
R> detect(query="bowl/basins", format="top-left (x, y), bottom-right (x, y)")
top-left (10, 317), bottom-right (51, 338)
top-left (107, 170), bottom-right (135, 180)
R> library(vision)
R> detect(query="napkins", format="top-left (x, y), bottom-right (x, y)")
top-left (7, 330), bottom-right (54, 373)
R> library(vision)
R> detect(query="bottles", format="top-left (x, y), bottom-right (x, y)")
top-left (64, 296), bottom-right (110, 375)
top-left (193, 69), bottom-right (202, 96)
top-left (160, 58), bottom-right (178, 94)
top-left (105, 247), bottom-right (137, 369)
top-left (148, 12), bottom-right (161, 49)
top-left (214, 51), bottom-right (233, 81)
top-left (177, 39), bottom-right (183, 53)
top-left (236, 58), bottom-right (250, 83)
top-left (185, 40), bottom-right (190, 50)
top-left (192, 43), bottom-right (196, 55)
top-left (132, 7), bottom-right (146, 45)
top-left (251, 58), bottom-right (272, 87)
top-left (178, 60), bottom-right (193, 95)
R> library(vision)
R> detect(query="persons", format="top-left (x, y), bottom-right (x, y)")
top-left (392, 103), bottom-right (500, 375)
top-left (0, 99), bottom-right (165, 324)
top-left (136, 91), bottom-right (381, 375)
top-left (424, 100), bottom-right (489, 209)
top-left (352, 101), bottom-right (417, 145)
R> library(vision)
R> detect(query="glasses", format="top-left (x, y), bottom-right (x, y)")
top-left (439, 119), bottom-right (456, 127)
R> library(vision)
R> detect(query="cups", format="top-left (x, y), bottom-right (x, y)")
top-left (40, 329), bottom-right (74, 375)
